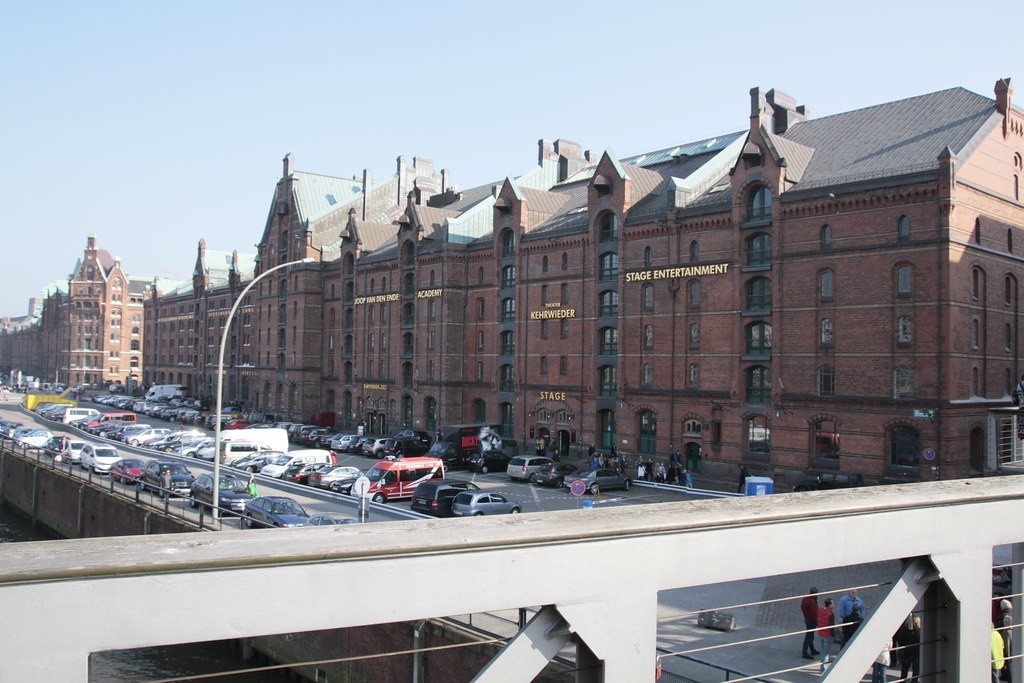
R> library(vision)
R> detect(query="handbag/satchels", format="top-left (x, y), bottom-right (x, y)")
top-left (663, 472), bottom-right (667, 480)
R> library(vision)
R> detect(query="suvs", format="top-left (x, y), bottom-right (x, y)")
top-left (450, 489), bottom-right (523, 517)
top-left (411, 479), bottom-right (481, 518)
top-left (191, 473), bottom-right (254, 516)
top-left (384, 431), bottom-right (433, 459)
top-left (139, 459), bottom-right (196, 498)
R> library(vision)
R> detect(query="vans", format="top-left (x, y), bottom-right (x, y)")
top-left (260, 450), bottom-right (332, 480)
top-left (88, 411), bottom-right (137, 432)
top-left (349, 455), bottom-right (445, 504)
top-left (221, 442), bottom-right (272, 466)
top-left (506, 454), bottom-right (557, 482)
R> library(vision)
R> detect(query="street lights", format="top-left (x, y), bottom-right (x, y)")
top-left (210, 257), bottom-right (317, 526)
top-left (179, 329), bottom-right (212, 405)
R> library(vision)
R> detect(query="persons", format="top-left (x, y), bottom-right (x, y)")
top-left (841, 604), bottom-right (864, 644)
top-left (225, 399), bottom-right (263, 412)
top-left (738, 463), bottom-right (750, 493)
top-left (535, 436), bottom-right (693, 488)
top-left (480, 426), bottom-right (502, 451)
top-left (872, 637), bottom-right (893, 683)
top-left (887, 591), bottom-right (1012, 683)
top-left (816, 598), bottom-right (835, 673)
top-left (800, 587), bottom-right (819, 659)
top-left (838, 589), bottom-right (865, 619)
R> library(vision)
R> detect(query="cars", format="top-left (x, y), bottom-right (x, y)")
top-left (242, 496), bottom-right (359, 528)
top-left (534, 462), bottom-right (631, 495)
top-left (0, 375), bottom-right (67, 393)
top-left (37, 404), bottom-right (215, 461)
top-left (283, 462), bottom-right (368, 492)
top-left (78, 383), bottom-right (392, 459)
top-left (111, 459), bottom-right (145, 484)
top-left (0, 419), bottom-right (123, 473)
top-left (462, 450), bottom-right (511, 473)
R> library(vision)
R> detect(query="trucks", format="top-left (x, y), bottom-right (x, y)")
top-left (421, 421), bottom-right (503, 472)
top-left (24, 387), bottom-right (77, 410)
top-left (220, 428), bottom-right (288, 463)
top-left (145, 384), bottom-right (182, 402)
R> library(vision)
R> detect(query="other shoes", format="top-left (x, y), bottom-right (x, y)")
top-left (803, 655), bottom-right (815, 659)
top-left (811, 651), bottom-right (819, 655)
